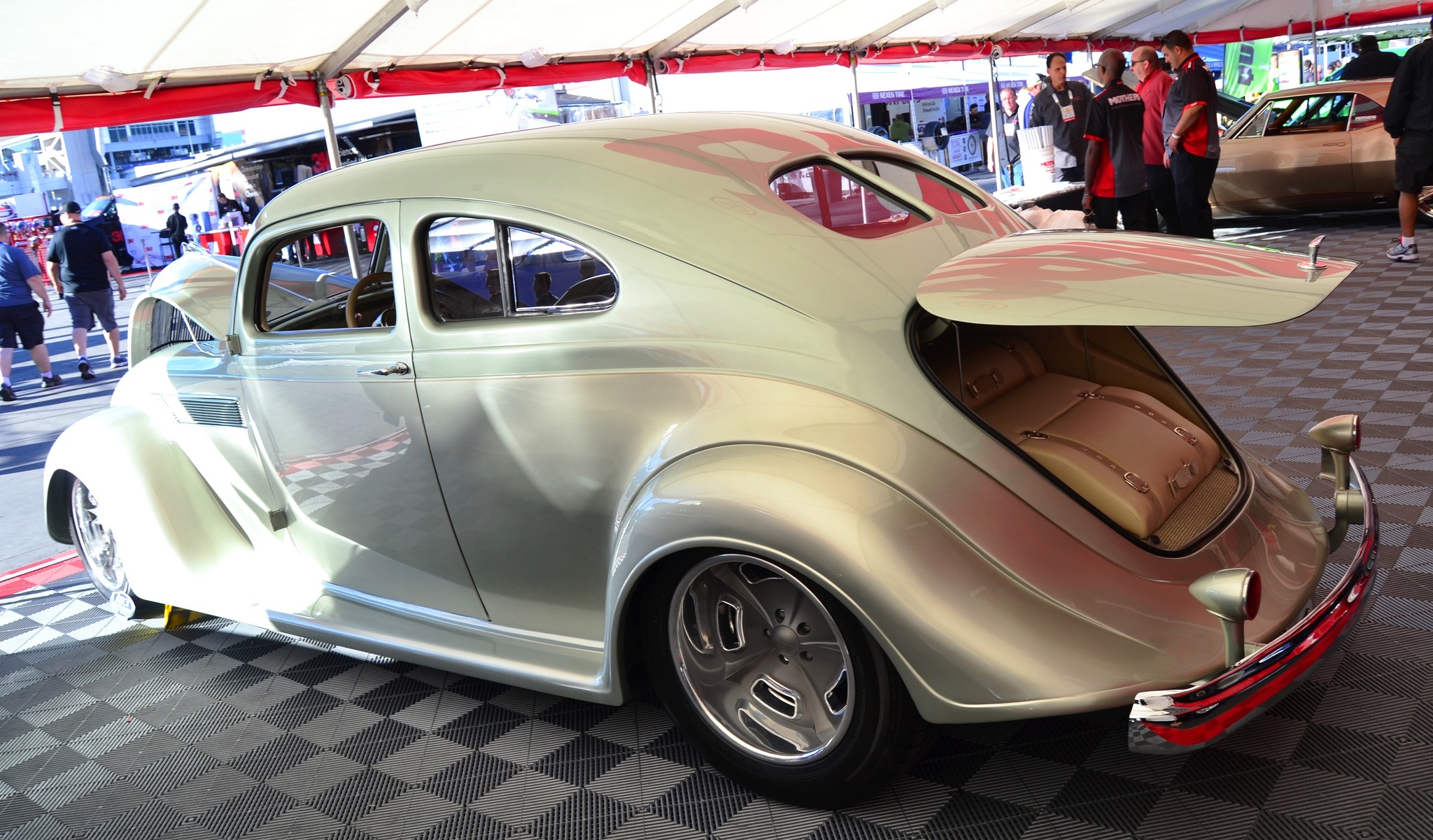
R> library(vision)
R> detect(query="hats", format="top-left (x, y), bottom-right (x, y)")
top-left (1026, 73), bottom-right (1046, 88)
top-left (54, 201), bottom-right (81, 215)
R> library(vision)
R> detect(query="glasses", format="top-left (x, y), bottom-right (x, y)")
top-left (972, 108), bottom-right (978, 111)
top-left (1132, 60), bottom-right (1145, 67)
top-left (1093, 63), bottom-right (1103, 68)
top-left (985, 96), bottom-right (987, 98)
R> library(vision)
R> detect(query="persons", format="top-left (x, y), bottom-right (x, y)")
top-left (166, 203), bottom-right (189, 259)
top-left (891, 113), bottom-right (914, 143)
top-left (1027, 52), bottom-right (1096, 182)
top-left (217, 192), bottom-right (243, 225)
top-left (580, 253), bottom-right (595, 282)
top-left (1016, 73), bottom-right (1052, 130)
top-left (532, 272), bottom-right (559, 307)
top-left (1160, 29), bottom-right (1221, 240)
top-left (984, 93), bottom-right (1000, 113)
top-left (0, 222), bottom-right (62, 401)
top-left (1079, 48), bottom-right (1151, 231)
top-left (1383, 14), bottom-right (1433, 260)
top-left (985, 87), bottom-right (1024, 188)
top-left (1341, 34), bottom-right (1401, 81)
top-left (1129, 46), bottom-right (1184, 236)
top-left (485, 269), bottom-right (504, 308)
top-left (1244, 52), bottom-right (1343, 103)
top-left (959, 103), bottom-right (982, 172)
top-left (45, 201), bottom-right (128, 379)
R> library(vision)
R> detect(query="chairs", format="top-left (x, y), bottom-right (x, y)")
top-left (557, 274), bottom-right (615, 306)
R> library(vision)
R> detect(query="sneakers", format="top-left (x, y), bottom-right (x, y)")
top-left (78, 356), bottom-right (95, 379)
top-left (0, 383), bottom-right (18, 401)
top-left (110, 355), bottom-right (128, 368)
top-left (41, 374), bottom-right (62, 388)
top-left (1386, 237), bottom-right (1419, 260)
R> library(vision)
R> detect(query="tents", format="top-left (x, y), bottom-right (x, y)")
top-left (1, 1), bottom-right (1431, 278)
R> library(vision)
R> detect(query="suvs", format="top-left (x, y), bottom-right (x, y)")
top-left (78, 195), bottom-right (134, 265)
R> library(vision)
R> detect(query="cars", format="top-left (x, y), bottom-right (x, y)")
top-left (41, 109), bottom-right (1381, 808)
top-left (1080, 67), bottom-right (1433, 225)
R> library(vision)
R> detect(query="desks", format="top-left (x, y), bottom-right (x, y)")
top-left (363, 221), bottom-right (390, 253)
top-left (234, 224), bottom-right (253, 256)
top-left (199, 228), bottom-right (234, 256)
top-left (305, 226), bottom-right (348, 259)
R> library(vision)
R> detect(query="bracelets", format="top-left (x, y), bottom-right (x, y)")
top-left (1172, 132), bottom-right (1184, 139)
top-left (1083, 193), bottom-right (1093, 196)
